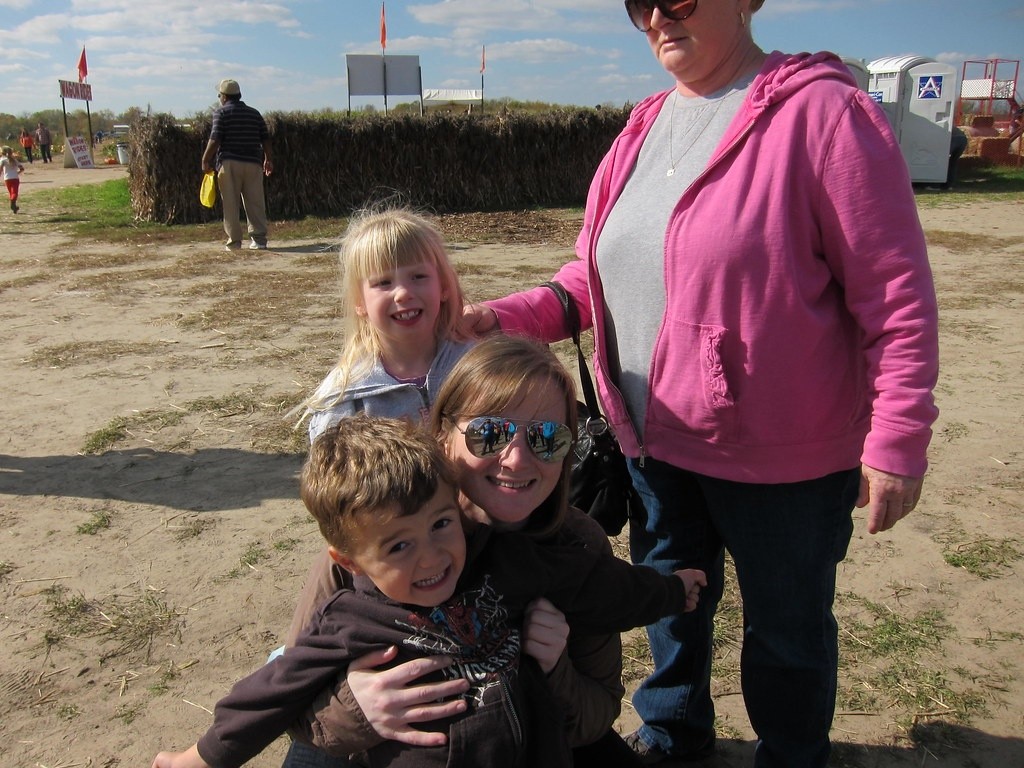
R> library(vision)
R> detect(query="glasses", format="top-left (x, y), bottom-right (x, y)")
top-left (625, 0), bottom-right (698, 31)
top-left (449, 414), bottom-right (574, 464)
top-left (218, 92), bottom-right (224, 98)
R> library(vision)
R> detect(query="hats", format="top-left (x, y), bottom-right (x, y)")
top-left (215, 79), bottom-right (240, 94)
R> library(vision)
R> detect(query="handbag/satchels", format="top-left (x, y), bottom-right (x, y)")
top-left (530, 430), bottom-right (536, 436)
top-left (536, 280), bottom-right (633, 536)
top-left (199, 169), bottom-right (216, 207)
top-left (494, 428), bottom-right (499, 434)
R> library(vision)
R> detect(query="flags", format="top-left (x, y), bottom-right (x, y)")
top-left (78, 49), bottom-right (87, 83)
top-left (381, 9), bottom-right (386, 47)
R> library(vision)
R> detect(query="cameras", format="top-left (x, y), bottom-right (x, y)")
top-left (487, 421), bottom-right (490, 423)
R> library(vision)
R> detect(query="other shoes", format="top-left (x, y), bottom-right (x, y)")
top-left (44, 160), bottom-right (47, 162)
top-left (543, 451), bottom-right (552, 460)
top-left (489, 450), bottom-right (495, 452)
top-left (11, 200), bottom-right (19, 213)
top-left (620, 726), bottom-right (716, 765)
top-left (481, 451), bottom-right (486, 455)
top-left (49, 157), bottom-right (52, 161)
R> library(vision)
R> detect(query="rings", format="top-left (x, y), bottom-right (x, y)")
top-left (903, 501), bottom-right (913, 507)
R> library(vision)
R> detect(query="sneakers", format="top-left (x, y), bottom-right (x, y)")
top-left (250, 241), bottom-right (265, 249)
top-left (225, 241), bottom-right (241, 251)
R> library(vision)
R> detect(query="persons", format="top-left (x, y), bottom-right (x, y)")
top-left (152, 417), bottom-right (707, 768)
top-left (202, 79), bottom-right (273, 250)
top-left (450, 0), bottom-right (939, 768)
top-left (96, 131), bottom-right (103, 143)
top-left (20, 123), bottom-right (52, 163)
top-left (281, 339), bottom-right (651, 768)
top-left (308, 214), bottom-right (474, 445)
top-left (466, 419), bottom-right (515, 456)
top-left (0, 147), bottom-right (24, 214)
top-left (529, 421), bottom-right (561, 461)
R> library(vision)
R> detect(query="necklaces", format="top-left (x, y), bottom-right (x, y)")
top-left (667, 52), bottom-right (762, 175)
